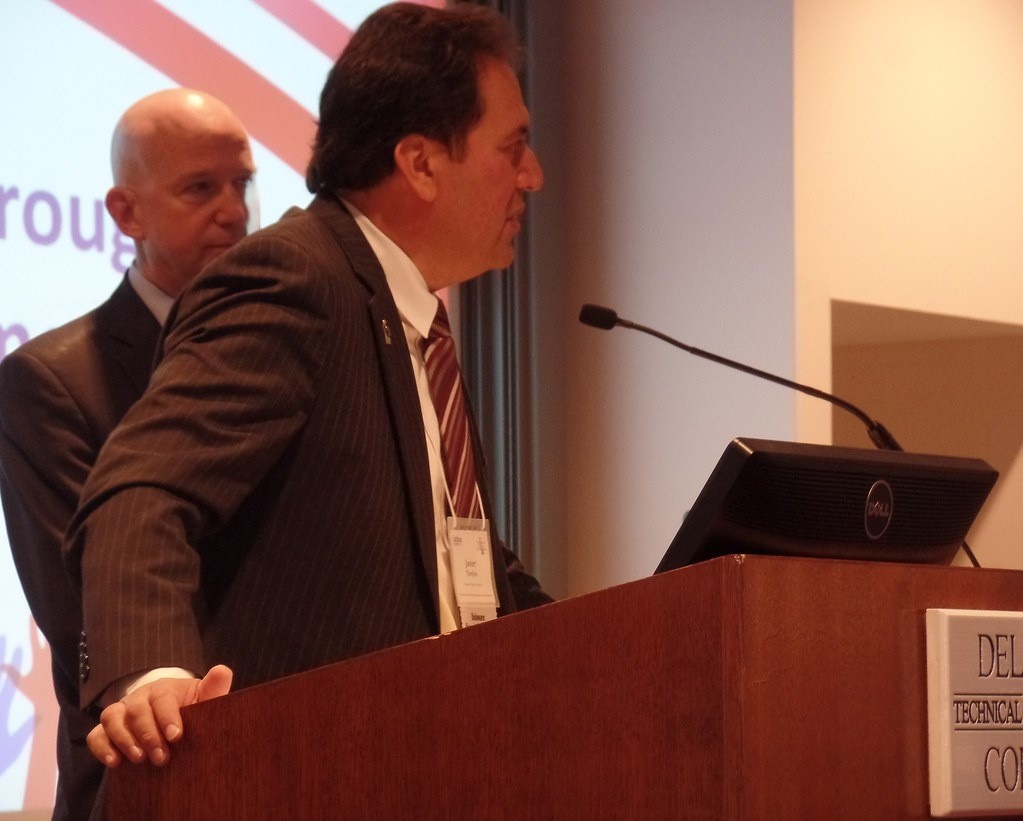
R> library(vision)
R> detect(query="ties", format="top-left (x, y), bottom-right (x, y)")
top-left (417, 297), bottom-right (487, 520)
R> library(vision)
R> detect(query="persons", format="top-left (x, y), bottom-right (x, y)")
top-left (74, 0), bottom-right (559, 770)
top-left (0, 89), bottom-right (262, 821)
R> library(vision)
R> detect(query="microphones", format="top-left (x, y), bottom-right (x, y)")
top-left (578, 304), bottom-right (903, 450)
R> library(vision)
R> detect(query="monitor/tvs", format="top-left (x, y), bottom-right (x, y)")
top-left (653, 437), bottom-right (998, 577)
